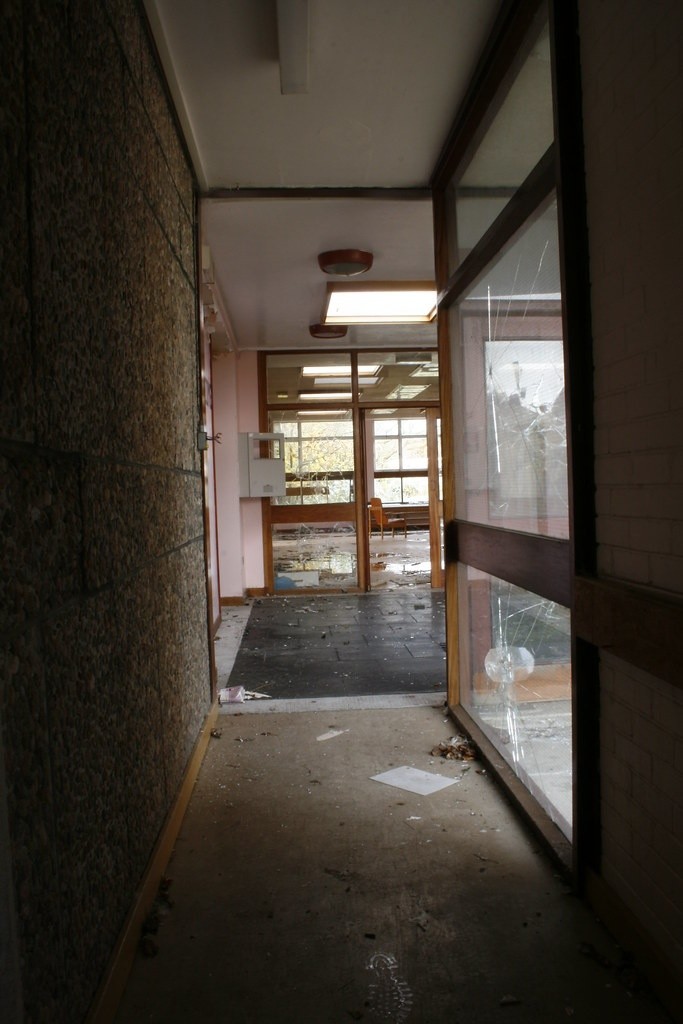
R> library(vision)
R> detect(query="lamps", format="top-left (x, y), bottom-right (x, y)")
top-left (318, 250), bottom-right (374, 276)
top-left (310, 324), bottom-right (347, 338)
top-left (321, 280), bottom-right (437, 326)
top-left (296, 365), bottom-right (385, 419)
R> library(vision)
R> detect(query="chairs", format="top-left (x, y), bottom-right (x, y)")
top-left (369, 498), bottom-right (406, 539)
